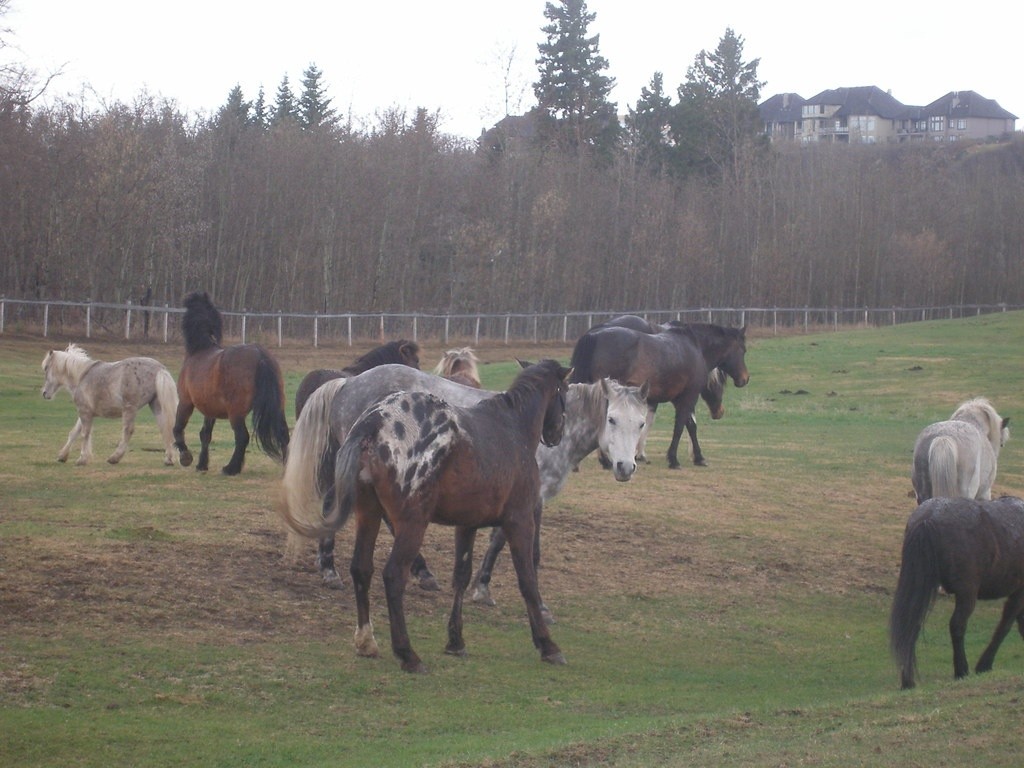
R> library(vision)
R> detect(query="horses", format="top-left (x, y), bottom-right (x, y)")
top-left (885, 496), bottom-right (1024, 690)
top-left (172, 290), bottom-right (291, 475)
top-left (277, 313), bottom-right (751, 675)
top-left (911, 396), bottom-right (1010, 596)
top-left (41, 342), bottom-right (180, 468)
